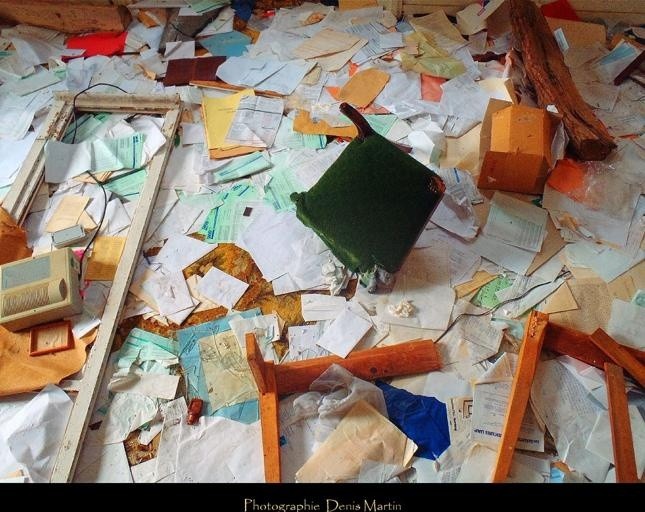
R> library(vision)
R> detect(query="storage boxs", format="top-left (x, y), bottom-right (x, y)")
top-left (476, 97), bottom-right (557, 195)
